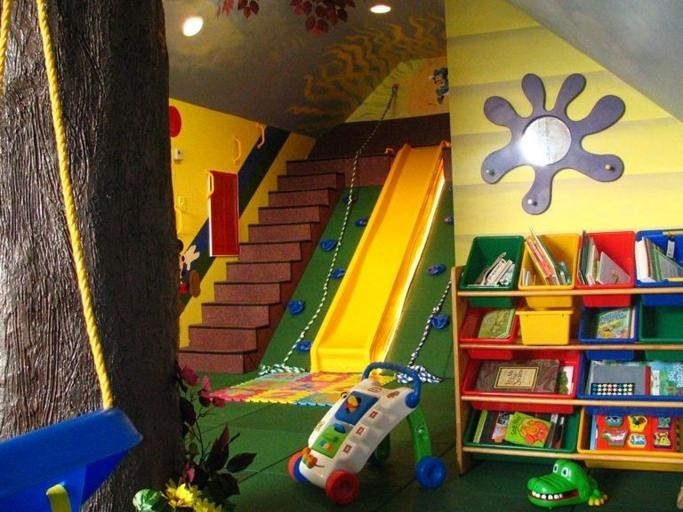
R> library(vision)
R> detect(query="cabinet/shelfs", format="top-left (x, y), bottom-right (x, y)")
top-left (450, 267), bottom-right (683, 479)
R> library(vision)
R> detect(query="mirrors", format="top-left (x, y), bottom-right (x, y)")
top-left (463, 71), bottom-right (625, 215)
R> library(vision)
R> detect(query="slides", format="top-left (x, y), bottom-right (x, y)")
top-left (311, 142), bottom-right (446, 374)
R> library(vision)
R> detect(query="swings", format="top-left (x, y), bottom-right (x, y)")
top-left (2, 0), bottom-right (142, 511)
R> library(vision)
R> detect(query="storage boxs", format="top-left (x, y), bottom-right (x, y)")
top-left (459, 228), bottom-right (683, 470)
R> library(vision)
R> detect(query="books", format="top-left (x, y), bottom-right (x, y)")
top-left (472, 410), bottom-right (566, 449)
top-left (475, 228), bottom-right (683, 339)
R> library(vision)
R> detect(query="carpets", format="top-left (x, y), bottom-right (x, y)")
top-left (209, 372), bottom-right (396, 407)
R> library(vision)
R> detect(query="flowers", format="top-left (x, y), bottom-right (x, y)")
top-left (132, 357), bottom-right (256, 510)
